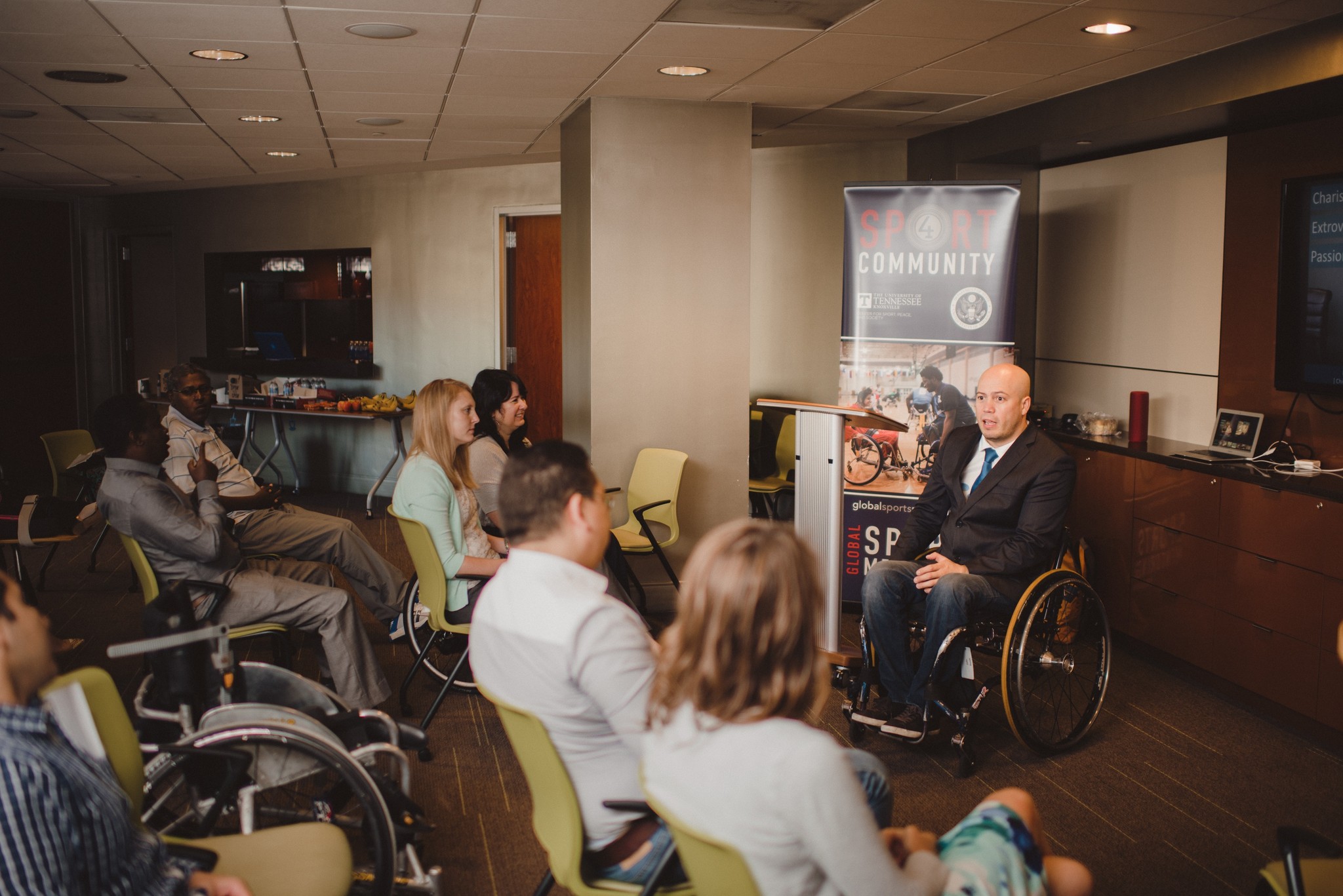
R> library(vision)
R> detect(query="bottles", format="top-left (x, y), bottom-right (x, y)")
top-left (293, 376), bottom-right (326, 394)
top-left (283, 380), bottom-right (292, 396)
top-left (348, 340), bottom-right (370, 365)
top-left (270, 379), bottom-right (279, 408)
top-left (353, 270), bottom-right (369, 298)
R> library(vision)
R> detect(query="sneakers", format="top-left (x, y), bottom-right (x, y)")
top-left (388, 607), bottom-right (431, 640)
top-left (881, 705), bottom-right (940, 739)
top-left (412, 589), bottom-right (420, 605)
top-left (891, 457), bottom-right (909, 467)
top-left (850, 695), bottom-right (894, 727)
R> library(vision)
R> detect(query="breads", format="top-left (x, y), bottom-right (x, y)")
top-left (1087, 417), bottom-right (1118, 435)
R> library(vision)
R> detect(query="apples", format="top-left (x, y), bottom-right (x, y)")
top-left (337, 400), bottom-right (361, 412)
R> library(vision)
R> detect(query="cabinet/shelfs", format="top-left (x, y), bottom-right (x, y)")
top-left (1046, 427), bottom-right (1343, 758)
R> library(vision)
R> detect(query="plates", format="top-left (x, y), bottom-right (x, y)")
top-left (225, 394), bottom-right (229, 404)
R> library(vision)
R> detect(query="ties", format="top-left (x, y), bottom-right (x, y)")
top-left (970, 448), bottom-right (998, 493)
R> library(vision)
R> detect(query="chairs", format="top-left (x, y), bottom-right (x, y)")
top-left (746, 413), bottom-right (798, 523)
top-left (472, 683), bottom-right (762, 896)
top-left (389, 503), bottom-right (472, 734)
top-left (36, 668), bottom-right (352, 894)
top-left (602, 449), bottom-right (688, 606)
top-left (38, 430), bottom-right (140, 594)
top-left (118, 527), bottom-right (289, 663)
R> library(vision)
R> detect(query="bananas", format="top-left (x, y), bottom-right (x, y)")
top-left (397, 390), bottom-right (417, 409)
top-left (361, 392), bottom-right (397, 411)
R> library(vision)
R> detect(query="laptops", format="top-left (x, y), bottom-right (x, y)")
top-left (1174, 409), bottom-right (1264, 462)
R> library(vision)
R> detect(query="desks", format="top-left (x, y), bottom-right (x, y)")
top-left (238, 404), bottom-right (415, 520)
top-left (146, 396), bottom-right (283, 499)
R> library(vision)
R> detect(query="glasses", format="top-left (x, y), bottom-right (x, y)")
top-left (171, 385), bottom-right (214, 396)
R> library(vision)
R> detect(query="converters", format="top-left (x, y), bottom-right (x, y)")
top-left (1294, 459), bottom-right (1320, 469)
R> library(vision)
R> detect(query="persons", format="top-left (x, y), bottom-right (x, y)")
top-left (1, 366), bottom-right (890, 896)
top-left (642, 519), bottom-right (1095, 896)
top-left (852, 363), bottom-right (1073, 739)
top-left (843, 367), bottom-right (978, 468)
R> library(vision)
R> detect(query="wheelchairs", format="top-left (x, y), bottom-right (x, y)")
top-left (906, 402), bottom-right (935, 431)
top-left (844, 426), bottom-right (915, 485)
top-left (829, 525), bottom-right (1113, 779)
top-left (55, 622), bottom-right (446, 896)
top-left (404, 485), bottom-right (681, 696)
top-left (909, 432), bottom-right (940, 483)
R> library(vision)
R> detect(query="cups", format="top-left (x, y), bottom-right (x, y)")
top-left (215, 387), bottom-right (225, 405)
top-left (157, 372), bottom-right (162, 396)
top-left (1129, 392), bottom-right (1150, 443)
top-left (140, 379), bottom-right (150, 399)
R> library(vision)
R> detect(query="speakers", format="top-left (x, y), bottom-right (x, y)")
top-left (1274, 172), bottom-right (1343, 395)
